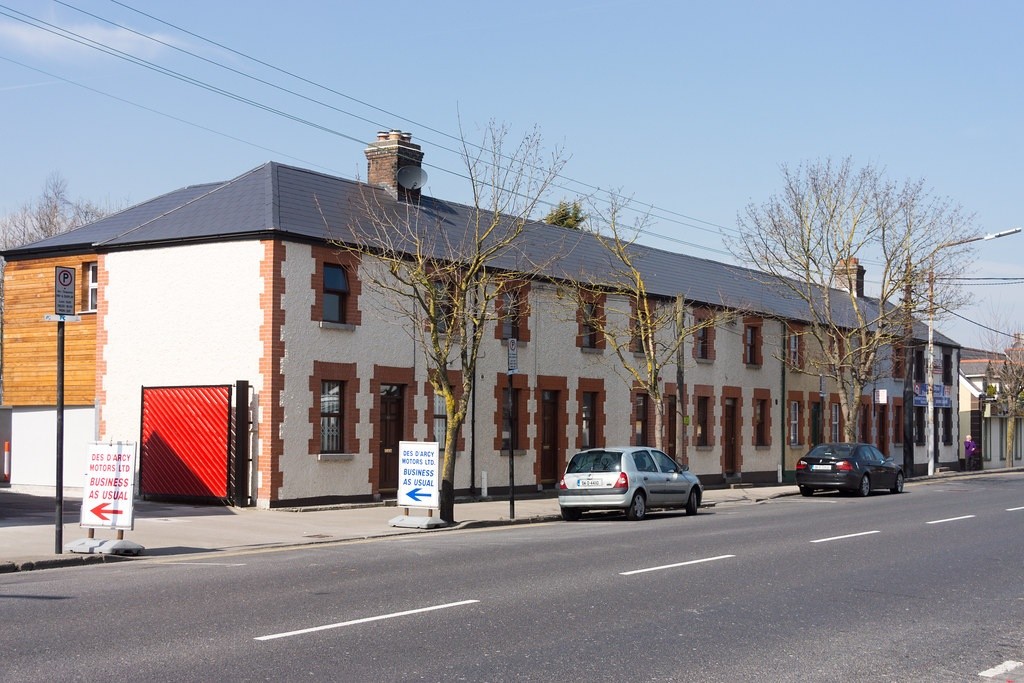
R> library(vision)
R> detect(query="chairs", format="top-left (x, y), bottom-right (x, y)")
top-left (635, 458), bottom-right (646, 471)
top-left (601, 458), bottom-right (614, 469)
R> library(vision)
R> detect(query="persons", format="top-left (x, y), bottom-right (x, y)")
top-left (963, 434), bottom-right (977, 471)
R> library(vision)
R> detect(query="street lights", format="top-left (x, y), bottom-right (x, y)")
top-left (924, 227), bottom-right (1023, 473)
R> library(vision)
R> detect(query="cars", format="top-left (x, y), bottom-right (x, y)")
top-left (559, 446), bottom-right (705, 521)
top-left (794, 441), bottom-right (906, 497)
